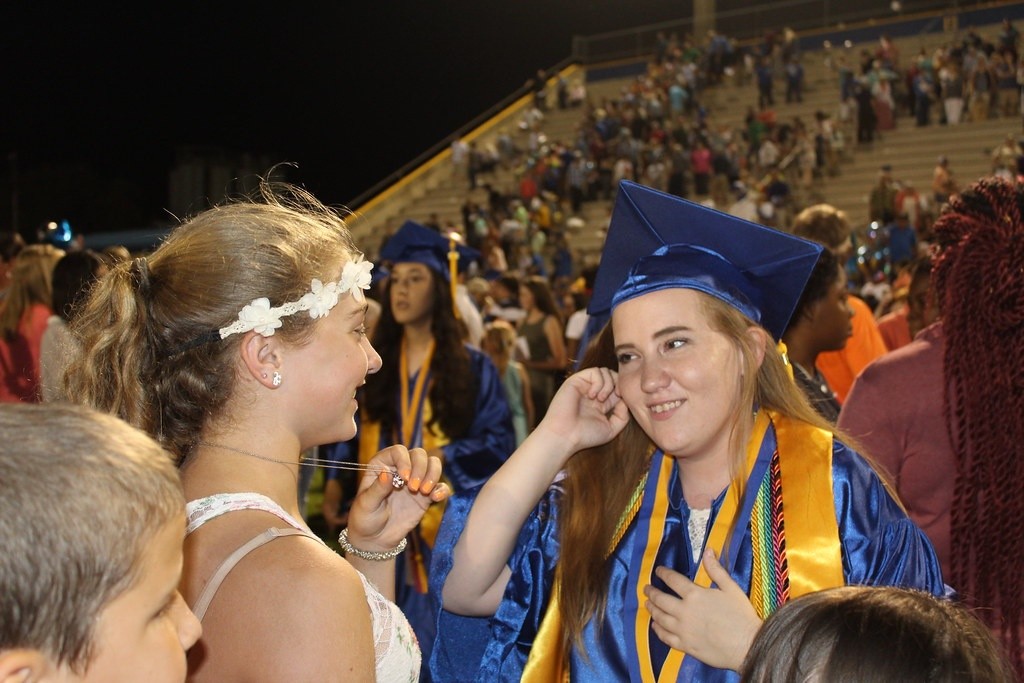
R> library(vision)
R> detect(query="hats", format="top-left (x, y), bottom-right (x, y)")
top-left (379, 219), bottom-right (480, 319)
top-left (586, 178), bottom-right (822, 386)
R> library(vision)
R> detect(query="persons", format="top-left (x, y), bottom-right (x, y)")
top-left (66, 198), bottom-right (454, 683)
top-left (776, 247), bottom-right (854, 426)
top-left (311, 217), bottom-right (517, 608)
top-left (735, 584), bottom-right (1016, 682)
top-left (415, 178), bottom-right (944, 683)
top-left (426, 181), bottom-right (610, 453)
top-left (754, 19), bottom-right (1024, 228)
top-left (0, 226), bottom-right (137, 411)
top-left (445, 25), bottom-right (766, 210)
top-left (790, 201), bottom-right (887, 409)
top-left (1, 398), bottom-right (203, 682)
top-left (835, 175), bottom-right (1023, 682)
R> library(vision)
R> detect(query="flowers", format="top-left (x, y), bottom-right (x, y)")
top-left (239, 297), bottom-right (283, 338)
top-left (296, 278), bottom-right (337, 320)
top-left (340, 254), bottom-right (374, 302)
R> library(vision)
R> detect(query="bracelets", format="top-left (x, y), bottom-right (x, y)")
top-left (338, 527), bottom-right (408, 561)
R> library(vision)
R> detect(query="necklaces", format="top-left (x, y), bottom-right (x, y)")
top-left (193, 438), bottom-right (403, 489)
